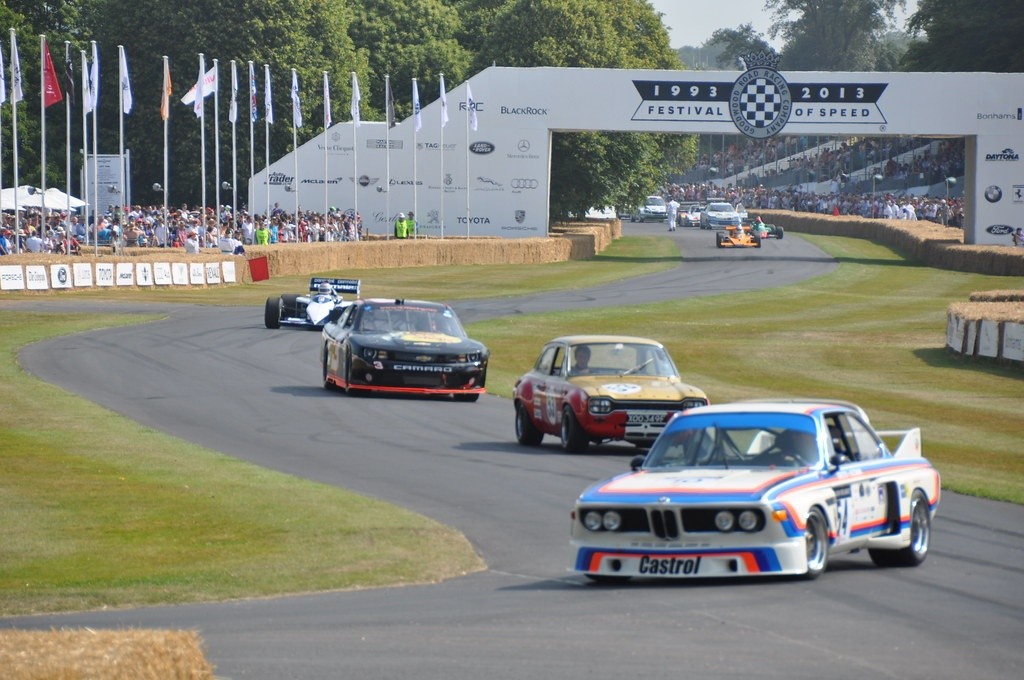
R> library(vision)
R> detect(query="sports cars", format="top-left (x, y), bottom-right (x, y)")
top-left (569, 398), bottom-right (943, 583)
top-left (321, 297), bottom-right (491, 403)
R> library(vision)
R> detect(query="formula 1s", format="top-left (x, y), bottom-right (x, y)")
top-left (265, 278), bottom-right (362, 328)
top-left (716, 225), bottom-right (762, 248)
top-left (749, 222), bottom-right (784, 240)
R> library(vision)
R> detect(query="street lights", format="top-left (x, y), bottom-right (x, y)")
top-left (871, 173), bottom-right (884, 218)
top-left (944, 177), bottom-right (957, 228)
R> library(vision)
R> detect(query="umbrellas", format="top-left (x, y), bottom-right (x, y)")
top-left (0, 186), bottom-right (89, 213)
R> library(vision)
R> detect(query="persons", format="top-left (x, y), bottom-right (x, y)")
top-left (394, 211), bottom-right (417, 239)
top-left (651, 134), bottom-right (966, 231)
top-left (0, 202), bottom-right (362, 256)
top-left (572, 345), bottom-right (590, 376)
top-left (1011, 228), bottom-right (1024, 246)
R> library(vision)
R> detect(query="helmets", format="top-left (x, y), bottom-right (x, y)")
top-left (317, 282), bottom-right (332, 296)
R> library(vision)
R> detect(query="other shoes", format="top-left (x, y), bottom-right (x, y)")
top-left (669, 228), bottom-right (672, 231)
top-left (673, 227), bottom-right (676, 231)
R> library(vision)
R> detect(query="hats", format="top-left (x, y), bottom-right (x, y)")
top-left (337, 208), bottom-right (341, 211)
top-left (5, 230), bottom-right (11, 235)
top-left (235, 230), bottom-right (242, 234)
top-left (330, 207), bottom-right (336, 211)
top-left (408, 212), bottom-right (414, 216)
top-left (226, 229), bottom-right (232, 234)
top-left (0, 227), bottom-right (6, 230)
top-left (399, 213), bottom-right (405, 218)
top-left (190, 231), bottom-right (198, 236)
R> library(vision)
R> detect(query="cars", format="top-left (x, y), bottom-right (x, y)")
top-left (512, 333), bottom-right (712, 456)
top-left (700, 202), bottom-right (748, 231)
top-left (618, 195), bottom-right (708, 226)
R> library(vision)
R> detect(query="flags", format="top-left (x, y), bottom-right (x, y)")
top-left (120, 49), bottom-right (132, 115)
top-left (264, 70), bottom-right (273, 126)
top-left (412, 81), bottom-right (422, 132)
top-left (181, 55), bottom-right (217, 117)
top-left (441, 75), bottom-right (449, 128)
top-left (228, 64), bottom-right (239, 122)
top-left (350, 76), bottom-right (364, 129)
top-left (387, 80), bottom-right (394, 132)
top-left (467, 81), bottom-right (477, 131)
top-left (250, 65), bottom-right (257, 124)
top-left (160, 64), bottom-right (173, 119)
top-left (41, 37), bottom-right (63, 110)
top-left (321, 74), bottom-right (331, 129)
top-left (0, 28), bottom-right (25, 109)
top-left (290, 72), bottom-right (303, 127)
top-left (63, 43), bottom-right (97, 112)
